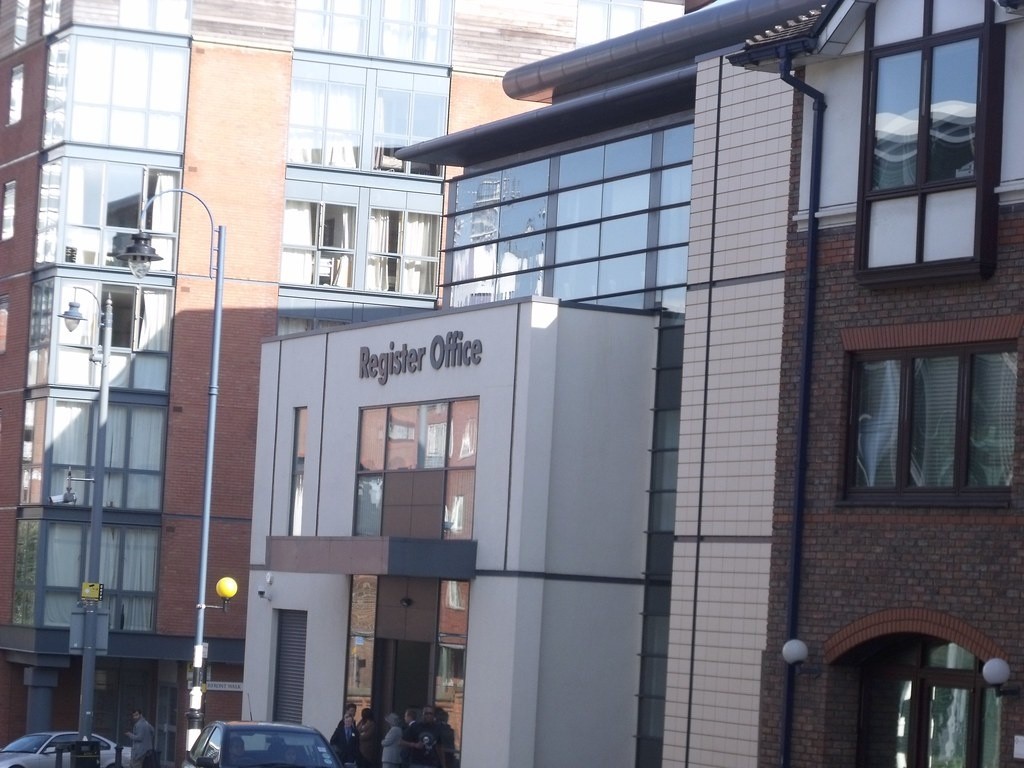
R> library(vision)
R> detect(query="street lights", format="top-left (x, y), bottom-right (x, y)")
top-left (60, 286), bottom-right (114, 740)
top-left (112, 189), bottom-right (240, 757)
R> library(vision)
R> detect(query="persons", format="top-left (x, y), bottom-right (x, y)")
top-left (228, 736), bottom-right (250, 767)
top-left (284, 744), bottom-right (298, 766)
top-left (401, 705), bottom-right (455, 768)
top-left (330, 713), bottom-right (358, 768)
top-left (125, 708), bottom-right (156, 768)
top-left (356, 708), bottom-right (377, 768)
top-left (339, 704), bottom-right (357, 728)
top-left (381, 713), bottom-right (404, 768)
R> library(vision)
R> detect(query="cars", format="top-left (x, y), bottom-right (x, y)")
top-left (178, 693), bottom-right (341, 768)
top-left (0, 728), bottom-right (136, 768)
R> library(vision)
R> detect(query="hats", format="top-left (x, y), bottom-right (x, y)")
top-left (384, 713), bottom-right (400, 726)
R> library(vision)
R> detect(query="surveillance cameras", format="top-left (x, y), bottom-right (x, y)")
top-left (257, 587), bottom-right (266, 598)
top-left (49, 493), bottom-right (77, 506)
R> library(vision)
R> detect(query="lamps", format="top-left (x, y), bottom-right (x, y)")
top-left (784, 640), bottom-right (820, 679)
top-left (205, 576), bottom-right (238, 614)
top-left (983, 657), bottom-right (1021, 697)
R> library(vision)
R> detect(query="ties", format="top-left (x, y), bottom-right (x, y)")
top-left (346, 729), bottom-right (350, 742)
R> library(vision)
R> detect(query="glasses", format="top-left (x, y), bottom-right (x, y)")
top-left (423, 712), bottom-right (433, 715)
